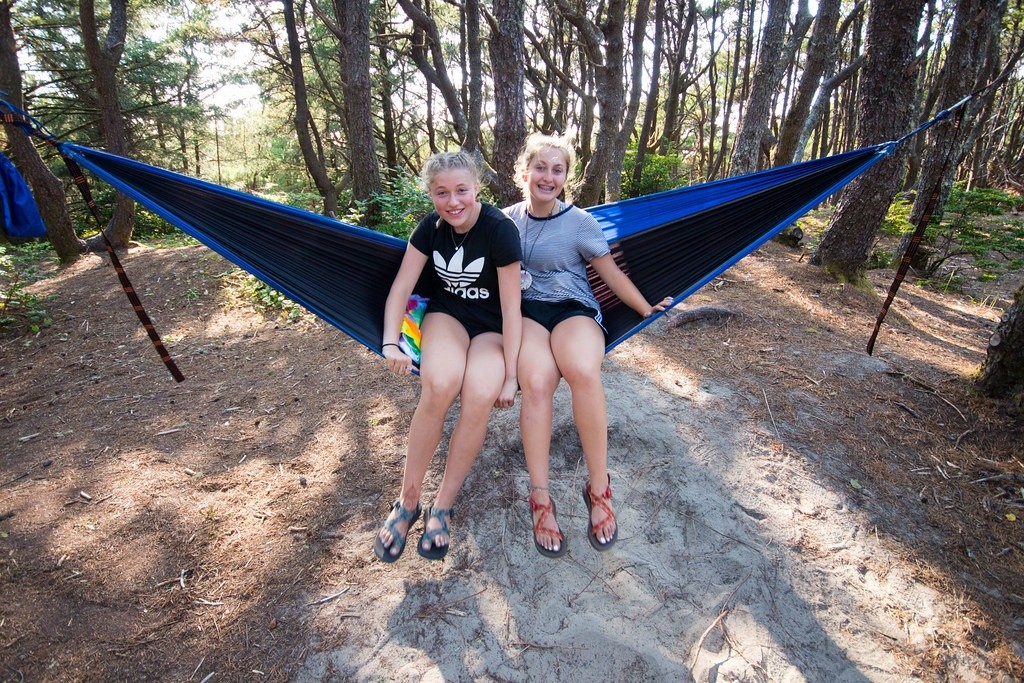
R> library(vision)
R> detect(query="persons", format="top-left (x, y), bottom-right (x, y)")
top-left (500, 133), bottom-right (675, 558)
top-left (373, 153), bottom-right (524, 564)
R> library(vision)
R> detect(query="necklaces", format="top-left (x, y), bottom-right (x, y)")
top-left (451, 204), bottom-right (476, 251)
top-left (518, 198), bottom-right (557, 291)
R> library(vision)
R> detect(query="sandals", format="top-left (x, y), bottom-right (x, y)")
top-left (417, 504), bottom-right (454, 560)
top-left (583, 480), bottom-right (618, 552)
top-left (530, 495), bottom-right (567, 558)
top-left (373, 500), bottom-right (422, 563)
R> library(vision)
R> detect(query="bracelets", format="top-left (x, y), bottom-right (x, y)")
top-left (382, 343), bottom-right (398, 348)
